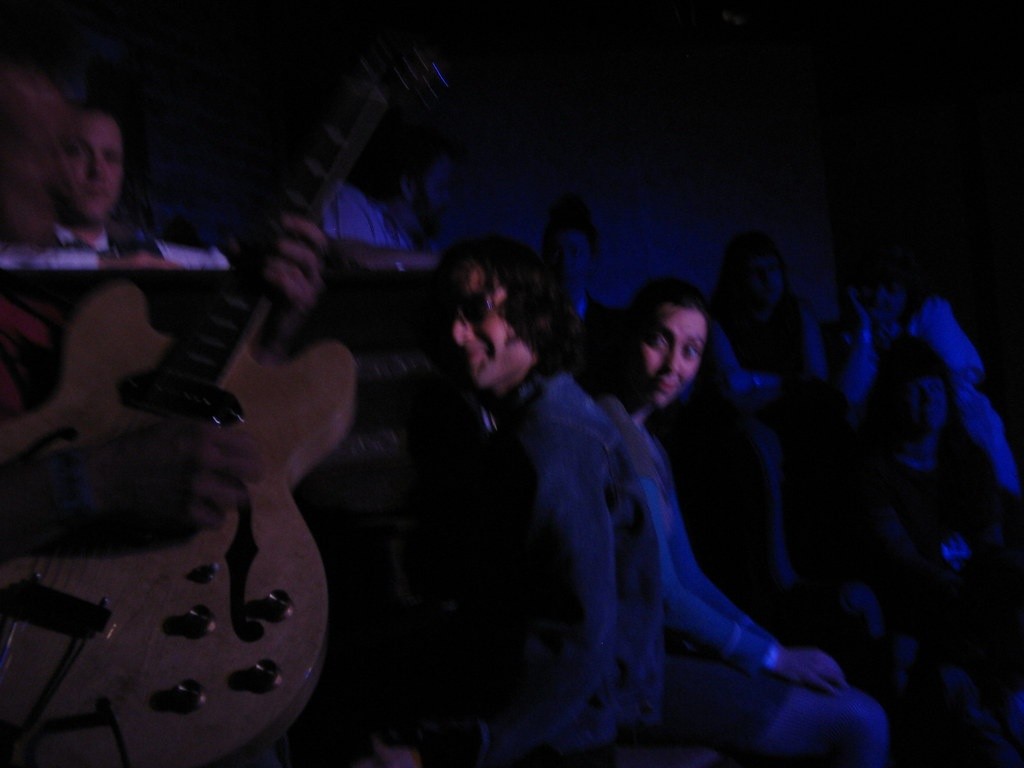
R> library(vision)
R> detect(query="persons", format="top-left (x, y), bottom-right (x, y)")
top-left (354, 237), bottom-right (666, 768)
top-left (647, 230), bottom-right (1022, 768)
top-left (320, 98), bottom-right (629, 406)
top-left (0, 97), bottom-right (234, 270)
top-left (587, 274), bottom-right (887, 766)
top-left (0, 64), bottom-right (332, 767)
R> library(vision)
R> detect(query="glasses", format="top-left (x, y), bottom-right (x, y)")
top-left (462, 293), bottom-right (490, 324)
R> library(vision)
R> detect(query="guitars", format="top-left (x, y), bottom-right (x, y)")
top-left (0, 44), bottom-right (443, 768)
